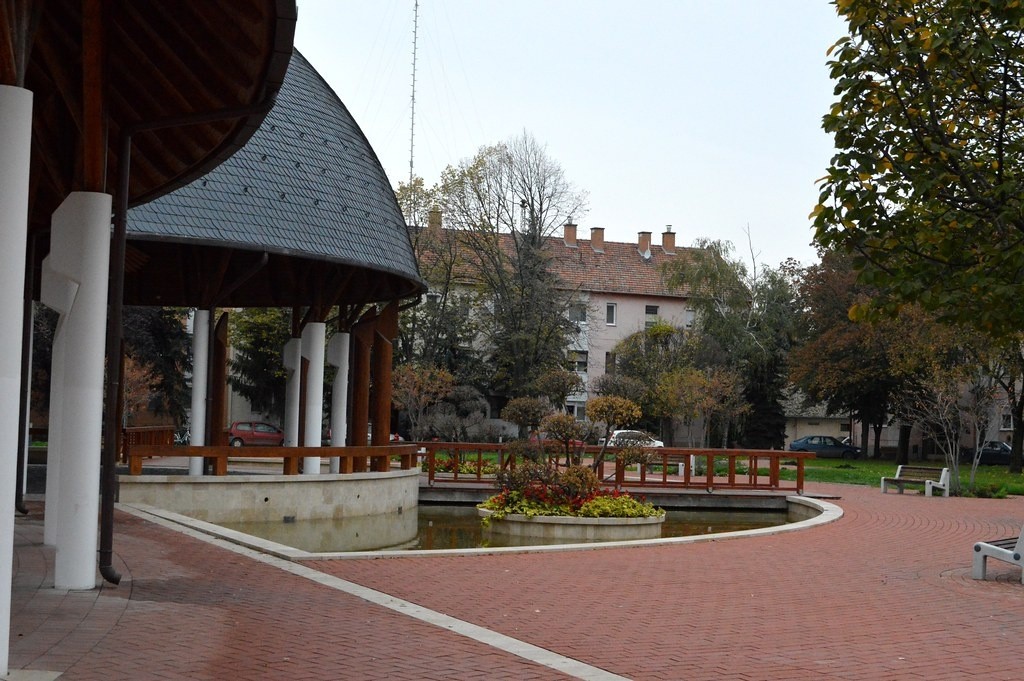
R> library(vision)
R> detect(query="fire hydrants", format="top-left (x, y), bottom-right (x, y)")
top-left (394, 433), bottom-right (399, 442)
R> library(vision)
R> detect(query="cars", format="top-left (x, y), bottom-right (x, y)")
top-left (529, 430), bottom-right (588, 447)
top-left (959, 440), bottom-right (1012, 465)
top-left (835, 436), bottom-right (850, 445)
top-left (228, 419), bottom-right (288, 451)
top-left (326, 422), bottom-right (405, 447)
top-left (788, 435), bottom-right (862, 460)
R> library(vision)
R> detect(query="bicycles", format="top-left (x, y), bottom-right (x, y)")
top-left (171, 426), bottom-right (192, 445)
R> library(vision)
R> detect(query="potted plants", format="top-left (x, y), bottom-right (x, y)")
top-left (474, 366), bottom-right (666, 541)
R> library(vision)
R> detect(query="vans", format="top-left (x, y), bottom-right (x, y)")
top-left (597, 429), bottom-right (663, 447)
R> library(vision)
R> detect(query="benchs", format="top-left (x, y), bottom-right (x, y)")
top-left (881, 465), bottom-right (950, 496)
top-left (973, 522), bottom-right (1024, 584)
